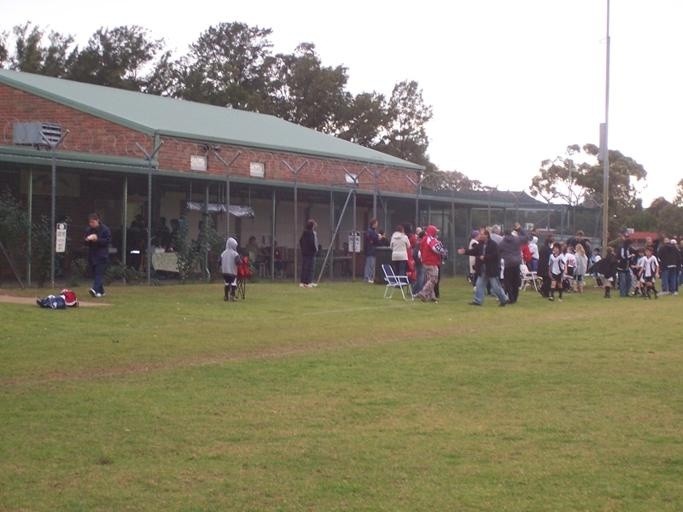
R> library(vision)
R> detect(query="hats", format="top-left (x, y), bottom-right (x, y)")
top-left (514, 223), bottom-right (521, 230)
top-left (471, 230), bottom-right (480, 238)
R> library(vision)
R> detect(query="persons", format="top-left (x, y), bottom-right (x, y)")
top-left (364, 220), bottom-right (382, 285)
top-left (390, 225), bottom-right (448, 305)
top-left (126, 214), bottom-right (204, 282)
top-left (53, 215), bottom-right (79, 285)
top-left (215, 237), bottom-right (240, 301)
top-left (300, 219), bottom-right (316, 288)
top-left (458, 221), bottom-right (592, 305)
top-left (268, 239), bottom-right (284, 274)
top-left (245, 237), bottom-right (270, 279)
top-left (379, 229), bottom-right (390, 247)
top-left (592, 230), bottom-right (683, 300)
top-left (81, 210), bottom-right (112, 298)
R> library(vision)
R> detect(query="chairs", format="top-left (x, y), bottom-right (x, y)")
top-left (379, 262), bottom-right (414, 302)
top-left (518, 262), bottom-right (539, 293)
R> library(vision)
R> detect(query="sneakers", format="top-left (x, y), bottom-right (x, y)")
top-left (299, 282), bottom-right (318, 288)
top-left (498, 299), bottom-right (511, 307)
top-left (655, 291), bottom-right (678, 297)
top-left (416, 293), bottom-right (427, 303)
top-left (89, 288), bottom-right (105, 297)
top-left (468, 301), bottom-right (481, 306)
top-left (430, 298), bottom-right (440, 304)
top-left (224, 295), bottom-right (235, 301)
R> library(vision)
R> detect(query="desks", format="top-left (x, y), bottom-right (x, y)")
top-left (150, 249), bottom-right (352, 280)
